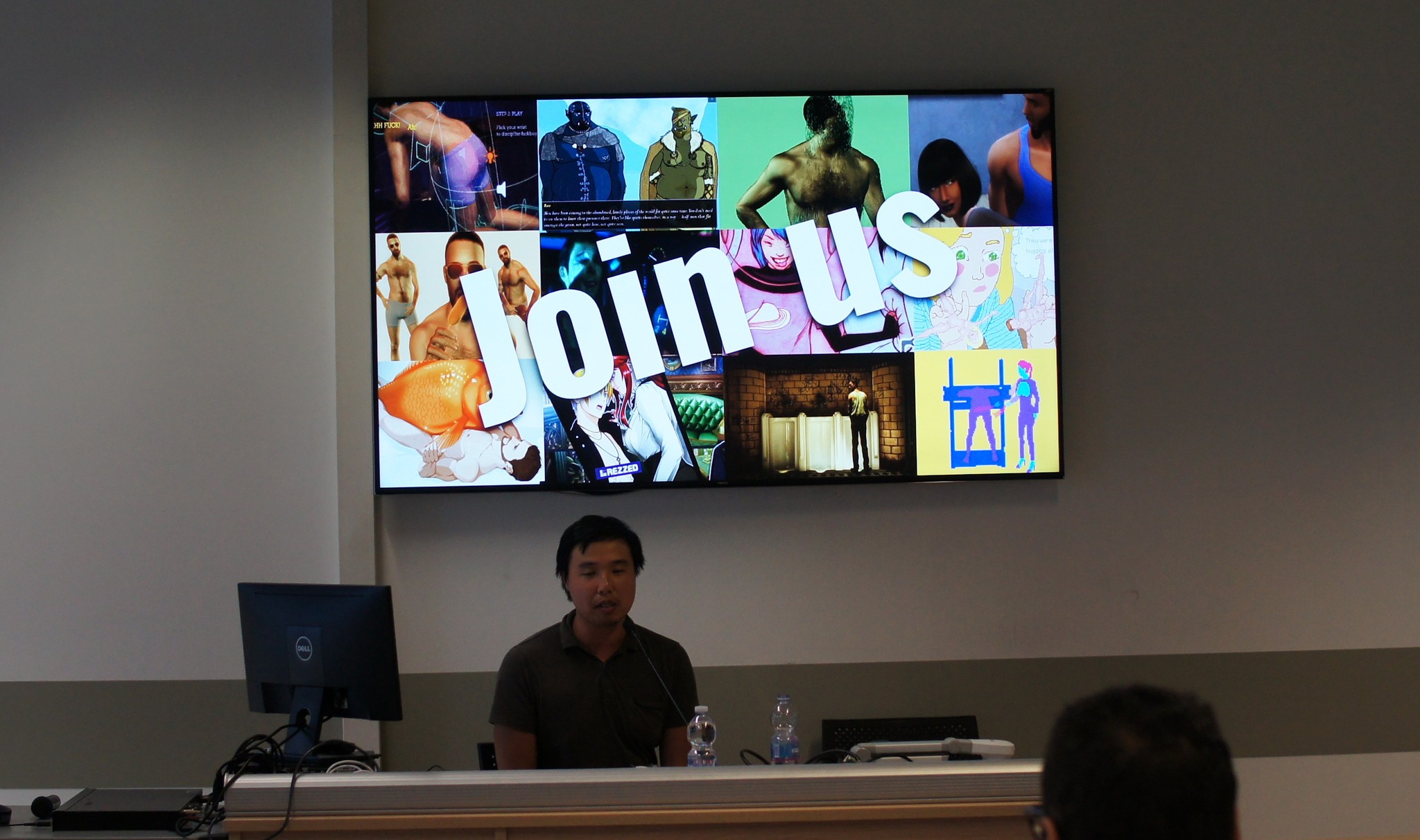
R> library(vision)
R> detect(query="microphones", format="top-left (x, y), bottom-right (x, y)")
top-left (31, 795), bottom-right (60, 818)
top-left (621, 618), bottom-right (688, 725)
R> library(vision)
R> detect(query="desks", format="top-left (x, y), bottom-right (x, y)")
top-left (220, 798), bottom-right (1043, 840)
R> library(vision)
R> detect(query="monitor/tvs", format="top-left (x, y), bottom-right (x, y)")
top-left (237, 581), bottom-right (402, 768)
top-left (368, 86), bottom-right (1064, 496)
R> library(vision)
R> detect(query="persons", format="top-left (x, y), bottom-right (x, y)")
top-left (410, 231), bottom-right (516, 362)
top-left (375, 234), bottom-right (419, 361)
top-left (489, 514), bottom-right (698, 768)
top-left (735, 93), bottom-right (885, 231)
top-left (987, 93), bottom-right (1054, 225)
top-left (849, 380), bottom-right (873, 475)
top-left (1027, 686), bottom-right (1244, 840)
top-left (497, 243), bottom-right (540, 321)
top-left (375, 98), bottom-right (539, 230)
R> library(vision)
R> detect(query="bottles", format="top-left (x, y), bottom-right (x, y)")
top-left (688, 706), bottom-right (717, 767)
top-left (771, 694), bottom-right (800, 765)
